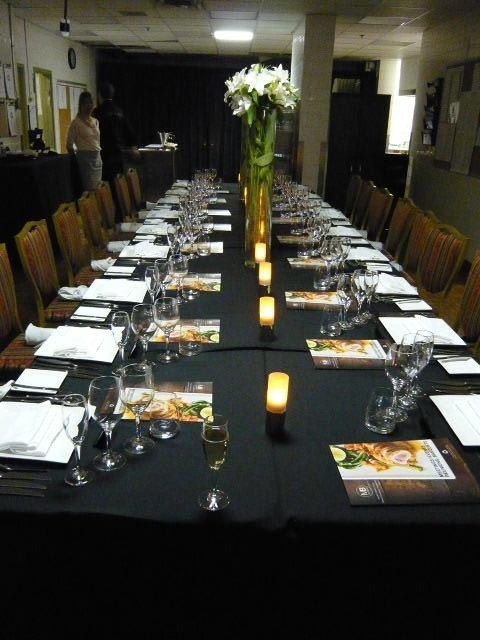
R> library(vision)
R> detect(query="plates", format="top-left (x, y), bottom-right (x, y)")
top-left (0, 178), bottom-right (191, 465)
top-left (297, 185), bottom-right (479, 448)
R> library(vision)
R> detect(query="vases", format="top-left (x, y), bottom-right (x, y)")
top-left (242, 106), bottom-right (276, 265)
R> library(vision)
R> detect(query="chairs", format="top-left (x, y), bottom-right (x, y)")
top-left (1, 167), bottom-right (154, 376)
top-left (343, 175), bottom-right (480, 362)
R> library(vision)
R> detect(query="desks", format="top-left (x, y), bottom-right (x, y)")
top-left (120, 143), bottom-right (180, 194)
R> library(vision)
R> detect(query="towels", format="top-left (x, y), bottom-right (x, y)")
top-left (172, 183), bottom-right (187, 187)
top-left (455, 394), bottom-right (480, 435)
top-left (146, 200), bottom-right (157, 210)
top-left (0, 400), bottom-right (70, 458)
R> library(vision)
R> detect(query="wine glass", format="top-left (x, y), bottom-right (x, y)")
top-left (64, 166), bottom-right (218, 487)
top-left (197, 415), bottom-right (230, 511)
top-left (274, 167), bottom-right (434, 424)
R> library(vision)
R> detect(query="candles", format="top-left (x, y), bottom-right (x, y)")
top-left (258, 261), bottom-right (272, 286)
top-left (258, 297), bottom-right (274, 326)
top-left (266, 372), bottom-right (289, 413)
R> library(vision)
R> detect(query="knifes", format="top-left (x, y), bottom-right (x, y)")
top-left (0, 465), bottom-right (50, 500)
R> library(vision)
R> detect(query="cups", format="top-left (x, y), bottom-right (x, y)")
top-left (272, 180), bottom-right (401, 434)
top-left (147, 177), bottom-right (223, 440)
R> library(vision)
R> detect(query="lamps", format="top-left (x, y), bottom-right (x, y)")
top-left (60, 0), bottom-right (70, 31)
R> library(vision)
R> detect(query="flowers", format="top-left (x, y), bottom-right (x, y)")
top-left (223, 62), bottom-right (301, 243)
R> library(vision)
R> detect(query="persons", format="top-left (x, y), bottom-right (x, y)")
top-left (90, 83), bottom-right (142, 185)
top-left (65, 91), bottom-right (103, 190)
